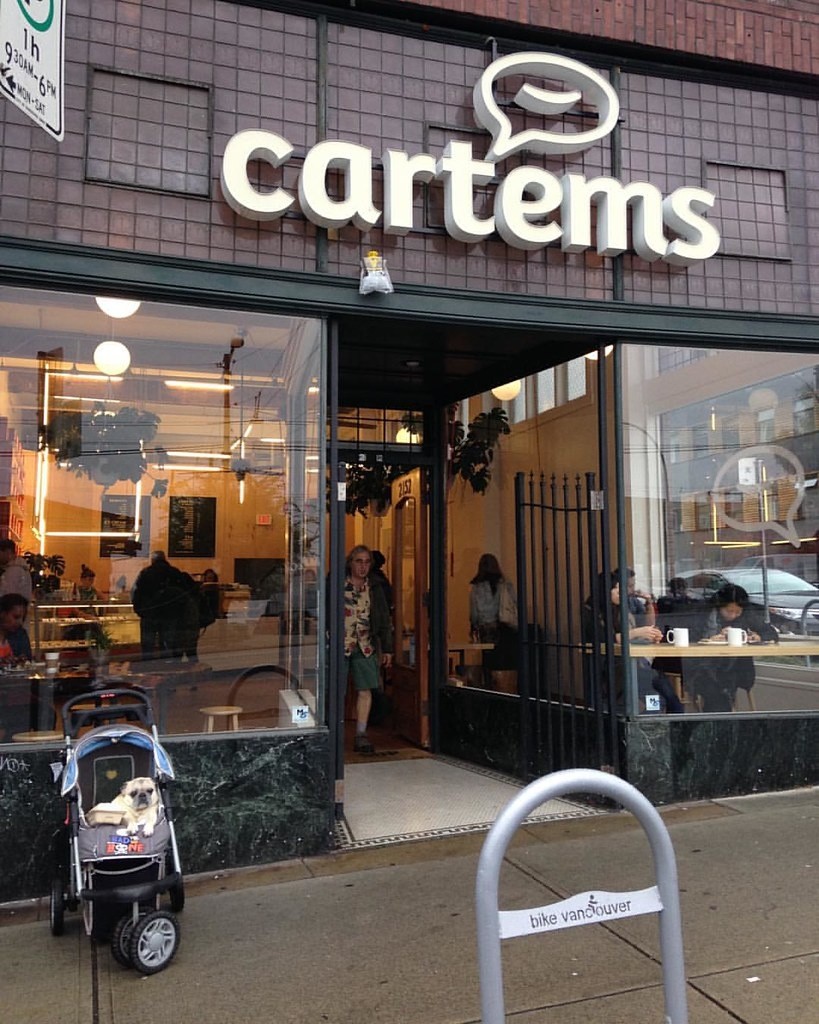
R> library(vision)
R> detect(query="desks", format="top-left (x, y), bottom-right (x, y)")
top-left (581, 642), bottom-right (819, 660)
top-left (400, 643), bottom-right (494, 665)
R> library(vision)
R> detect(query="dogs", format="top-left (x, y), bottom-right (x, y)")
top-left (111, 776), bottom-right (165, 836)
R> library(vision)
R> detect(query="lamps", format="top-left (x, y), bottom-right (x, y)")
top-left (747, 383), bottom-right (779, 412)
top-left (45, 294), bottom-right (614, 504)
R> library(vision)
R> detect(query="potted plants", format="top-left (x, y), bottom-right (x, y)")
top-left (327, 460), bottom-right (401, 516)
top-left (405, 404), bottom-right (510, 494)
top-left (44, 406), bottom-right (169, 496)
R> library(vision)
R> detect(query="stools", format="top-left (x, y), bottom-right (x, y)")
top-left (13, 730), bottom-right (64, 743)
top-left (70, 704), bottom-right (126, 736)
top-left (200, 705), bottom-right (243, 733)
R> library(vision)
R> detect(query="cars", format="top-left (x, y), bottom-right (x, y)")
top-left (666, 568), bottom-right (819, 634)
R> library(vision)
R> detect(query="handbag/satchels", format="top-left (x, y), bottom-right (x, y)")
top-left (497, 576), bottom-right (518, 628)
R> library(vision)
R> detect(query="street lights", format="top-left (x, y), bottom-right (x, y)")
top-left (219, 336), bottom-right (242, 453)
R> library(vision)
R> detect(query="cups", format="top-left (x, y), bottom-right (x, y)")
top-left (727, 628), bottom-right (748, 648)
top-left (666, 627), bottom-right (689, 648)
top-left (44, 653), bottom-right (60, 674)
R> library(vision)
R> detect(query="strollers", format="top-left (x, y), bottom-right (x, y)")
top-left (47, 686), bottom-right (192, 971)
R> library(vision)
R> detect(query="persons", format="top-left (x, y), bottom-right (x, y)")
top-left (682, 583), bottom-right (778, 711)
top-left (468, 554), bottom-right (518, 686)
top-left (62, 565), bottom-right (106, 650)
top-left (0, 538), bottom-right (32, 640)
top-left (580, 568), bottom-right (682, 713)
top-left (325, 545), bottom-right (392, 754)
top-left (640, 577), bottom-right (701, 713)
top-left (0, 593), bottom-right (32, 666)
top-left (131, 550), bottom-right (218, 663)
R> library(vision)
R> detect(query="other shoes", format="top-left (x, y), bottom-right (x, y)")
top-left (352, 742), bottom-right (374, 755)
